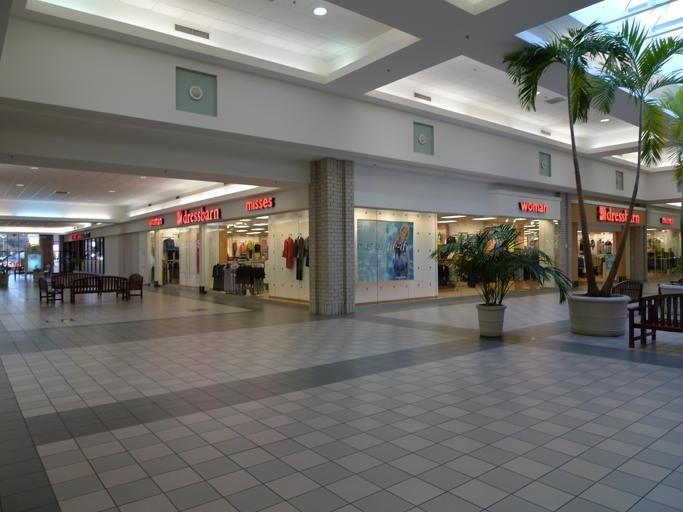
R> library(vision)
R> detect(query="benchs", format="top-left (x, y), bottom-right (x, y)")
top-left (38, 272), bottom-right (143, 304)
top-left (613, 280), bottom-right (683, 347)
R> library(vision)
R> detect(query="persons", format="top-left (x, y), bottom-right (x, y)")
top-left (392, 224), bottom-right (410, 280)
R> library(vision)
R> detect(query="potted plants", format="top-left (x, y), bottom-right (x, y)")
top-left (430, 224), bottom-right (576, 338)
